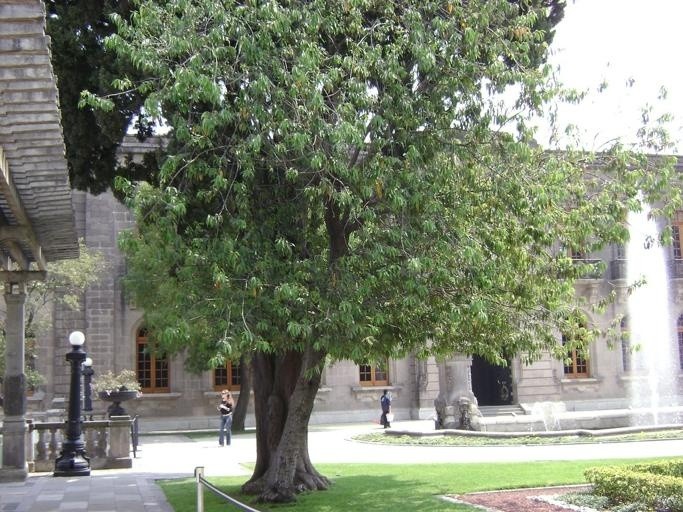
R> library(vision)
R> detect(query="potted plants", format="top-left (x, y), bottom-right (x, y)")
top-left (89, 367), bottom-right (143, 418)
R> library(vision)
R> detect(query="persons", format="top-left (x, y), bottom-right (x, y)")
top-left (380, 389), bottom-right (392, 429)
top-left (217, 390), bottom-right (234, 446)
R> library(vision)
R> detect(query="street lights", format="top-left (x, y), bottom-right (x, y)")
top-left (82, 355), bottom-right (93, 411)
top-left (50, 329), bottom-right (91, 478)
